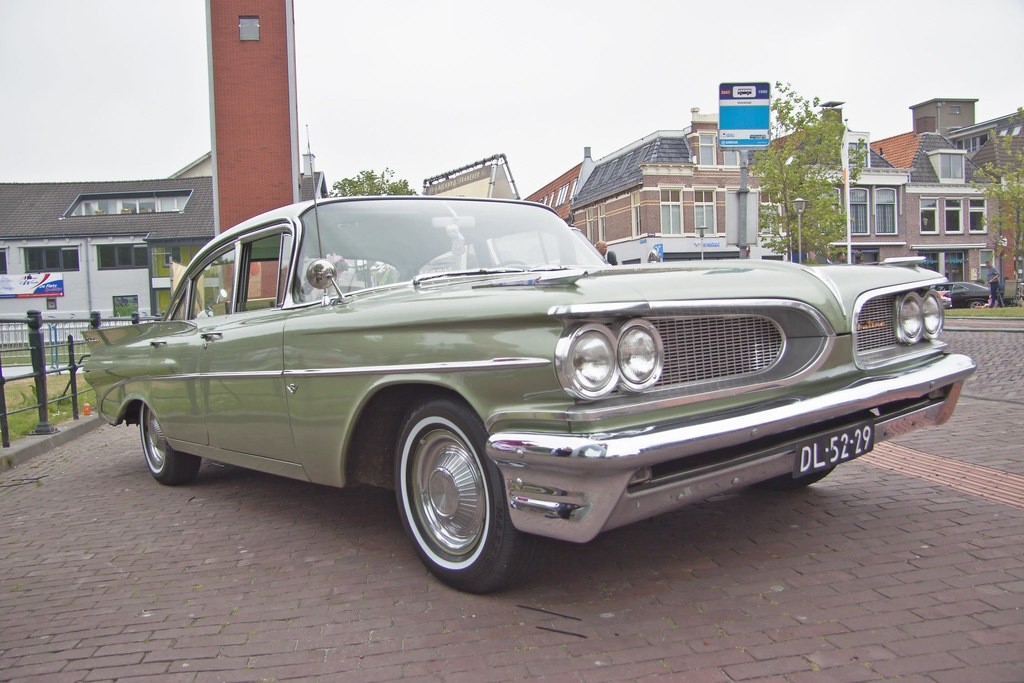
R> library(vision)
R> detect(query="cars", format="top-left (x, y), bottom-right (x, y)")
top-left (939, 282), bottom-right (990, 310)
top-left (75, 192), bottom-right (978, 599)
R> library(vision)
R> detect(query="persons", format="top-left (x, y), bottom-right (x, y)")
top-left (596, 241), bottom-right (607, 257)
top-left (922, 218), bottom-right (931, 231)
top-left (985, 261), bottom-right (1006, 307)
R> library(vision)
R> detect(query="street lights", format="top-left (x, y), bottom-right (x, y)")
top-left (791, 195), bottom-right (808, 263)
top-left (694, 226), bottom-right (708, 260)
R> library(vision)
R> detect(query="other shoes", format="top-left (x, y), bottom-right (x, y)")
top-left (989, 306), bottom-right (993, 308)
